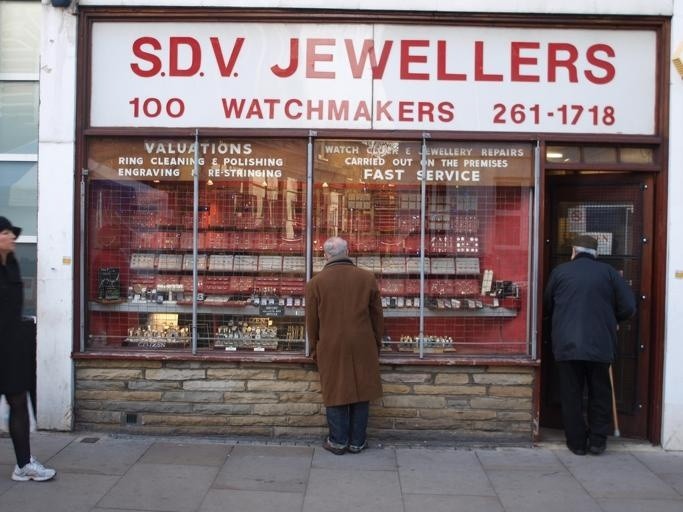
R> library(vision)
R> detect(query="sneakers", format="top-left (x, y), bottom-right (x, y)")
top-left (348, 440), bottom-right (368, 452)
top-left (325, 436), bottom-right (345, 455)
top-left (589, 442), bottom-right (605, 453)
top-left (11, 455), bottom-right (56, 482)
top-left (566, 440), bottom-right (586, 455)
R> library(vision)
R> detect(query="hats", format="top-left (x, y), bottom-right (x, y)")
top-left (571, 235), bottom-right (597, 251)
top-left (0, 216), bottom-right (21, 239)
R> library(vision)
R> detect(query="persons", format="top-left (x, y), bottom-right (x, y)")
top-left (0, 216), bottom-right (56, 482)
top-left (544, 235), bottom-right (635, 455)
top-left (305, 236), bottom-right (384, 455)
